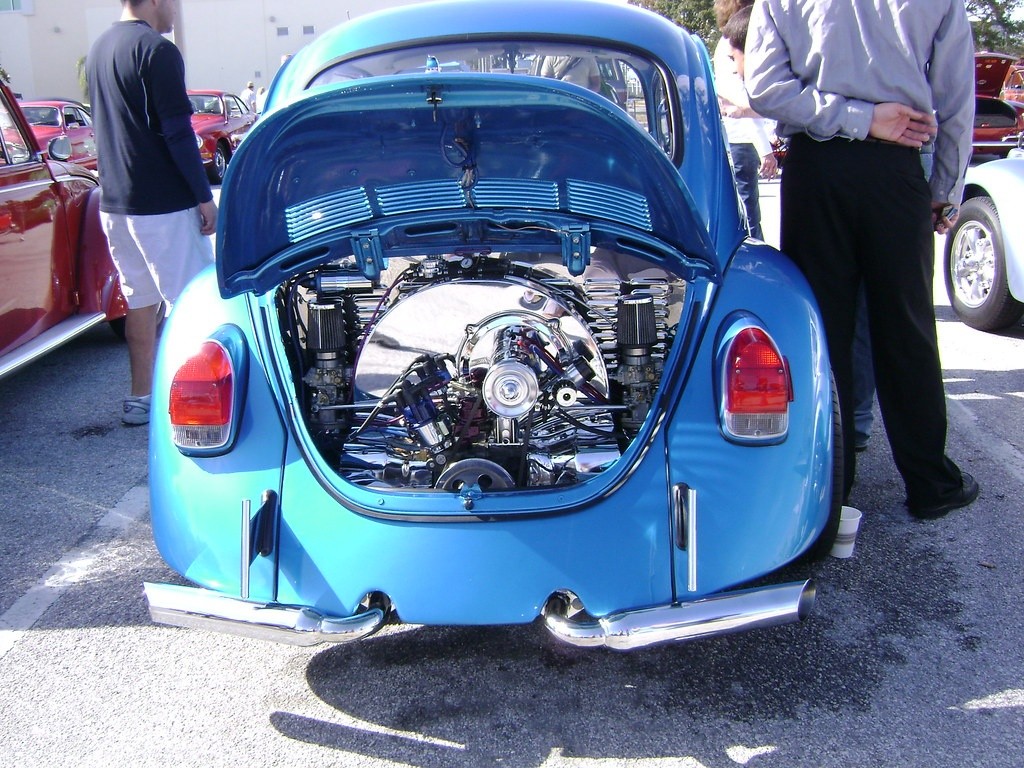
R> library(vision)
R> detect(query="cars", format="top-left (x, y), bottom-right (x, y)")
top-left (0, 68), bottom-right (165, 377)
top-left (938, 27), bottom-right (1024, 338)
top-left (0, 99), bottom-right (103, 174)
top-left (150, 0), bottom-right (839, 661)
top-left (181, 86), bottom-right (256, 183)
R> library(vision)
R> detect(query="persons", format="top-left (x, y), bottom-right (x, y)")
top-left (538, 48), bottom-right (600, 99)
top-left (82, 2), bottom-right (220, 427)
top-left (708, 1), bottom-right (984, 520)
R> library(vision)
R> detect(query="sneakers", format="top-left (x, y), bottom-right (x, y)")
top-left (854, 432), bottom-right (869, 447)
top-left (122, 394), bottom-right (151, 424)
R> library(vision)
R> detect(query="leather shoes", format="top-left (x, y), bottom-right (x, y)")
top-left (913, 472), bottom-right (979, 519)
